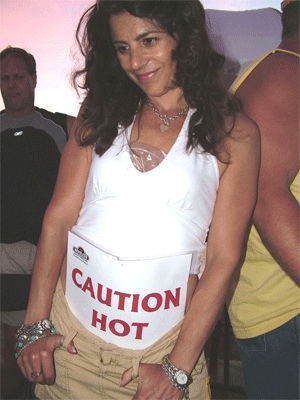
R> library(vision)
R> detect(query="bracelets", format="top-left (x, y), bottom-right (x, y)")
top-left (14, 319), bottom-right (56, 360)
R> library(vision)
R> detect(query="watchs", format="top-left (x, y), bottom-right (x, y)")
top-left (161, 355), bottom-right (194, 391)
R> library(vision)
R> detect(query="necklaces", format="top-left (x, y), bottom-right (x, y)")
top-left (146, 100), bottom-right (189, 127)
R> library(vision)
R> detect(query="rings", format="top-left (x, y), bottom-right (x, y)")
top-left (31, 370), bottom-right (42, 378)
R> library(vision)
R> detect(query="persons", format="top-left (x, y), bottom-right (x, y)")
top-left (1, 47), bottom-right (77, 399)
top-left (216, 1), bottom-right (300, 400)
top-left (24, 0), bottom-right (261, 399)
